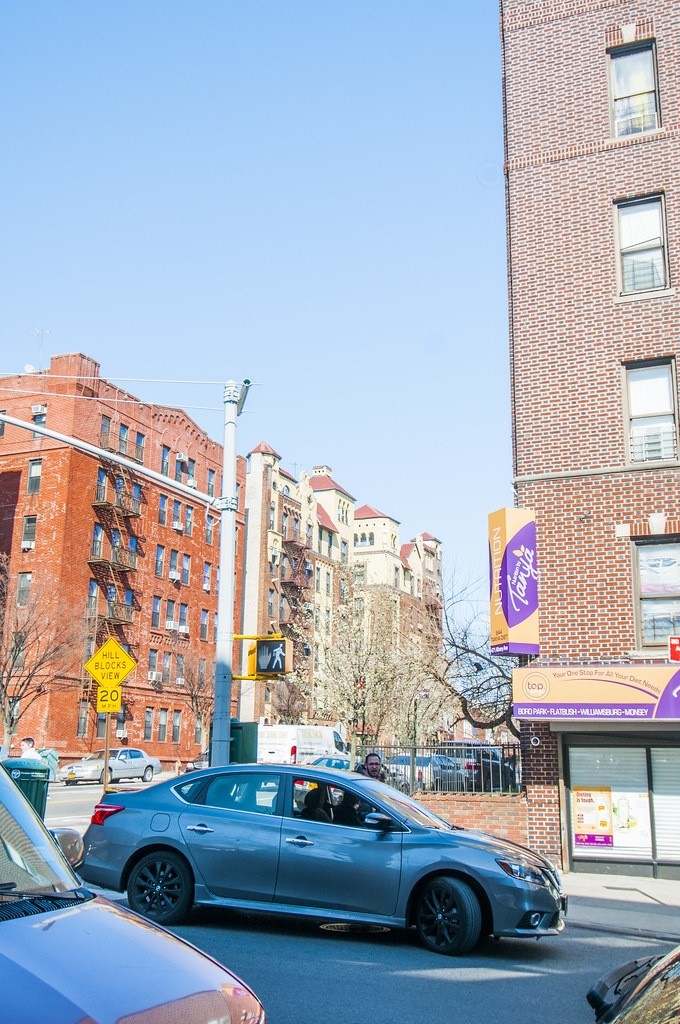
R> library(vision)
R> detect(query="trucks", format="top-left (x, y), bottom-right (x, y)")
top-left (258, 723), bottom-right (349, 768)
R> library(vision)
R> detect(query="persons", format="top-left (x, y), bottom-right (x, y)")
top-left (353, 753), bottom-right (386, 813)
top-left (332, 791), bottom-right (367, 828)
top-left (18, 738), bottom-right (42, 760)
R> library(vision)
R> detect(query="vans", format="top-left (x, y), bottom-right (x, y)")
top-left (436, 741), bottom-right (516, 789)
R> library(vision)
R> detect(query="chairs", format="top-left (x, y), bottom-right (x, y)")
top-left (301, 789), bottom-right (333, 823)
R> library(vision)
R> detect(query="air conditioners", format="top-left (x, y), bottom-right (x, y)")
top-left (20, 540), bottom-right (34, 549)
top-left (203, 584), bottom-right (210, 591)
top-left (32, 405), bottom-right (45, 415)
top-left (304, 602), bottom-right (314, 611)
top-left (169, 571), bottom-right (181, 581)
top-left (172, 522), bottom-right (183, 531)
top-left (176, 678), bottom-right (184, 685)
top-left (166, 621), bottom-right (179, 630)
top-left (176, 452), bottom-right (188, 462)
top-left (306, 563), bottom-right (313, 571)
top-left (117, 729), bottom-right (127, 738)
top-left (148, 671), bottom-right (162, 681)
top-left (179, 626), bottom-right (189, 633)
top-left (188, 479), bottom-right (198, 488)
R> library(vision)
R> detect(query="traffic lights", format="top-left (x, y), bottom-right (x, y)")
top-left (254, 638), bottom-right (293, 675)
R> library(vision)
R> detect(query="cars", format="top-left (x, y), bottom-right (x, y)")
top-left (0, 761), bottom-right (265, 1024)
top-left (586, 943), bottom-right (680, 1024)
top-left (57, 747), bottom-right (163, 787)
top-left (73, 764), bottom-right (569, 956)
top-left (185, 747), bottom-right (210, 772)
top-left (387, 753), bottom-right (470, 794)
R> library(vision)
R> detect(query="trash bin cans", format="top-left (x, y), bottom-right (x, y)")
top-left (38, 748), bottom-right (60, 782)
top-left (0, 758), bottom-right (51, 821)
